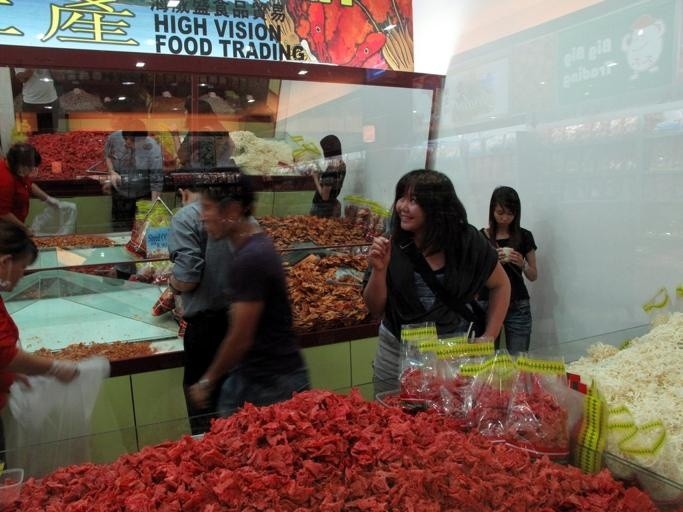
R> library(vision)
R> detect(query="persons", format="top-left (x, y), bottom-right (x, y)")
top-left (1, 141), bottom-right (62, 240)
top-left (104, 120), bottom-right (165, 279)
top-left (0, 219), bottom-right (80, 469)
top-left (186, 169), bottom-right (310, 420)
top-left (165, 174), bottom-right (235, 435)
top-left (178, 98), bottom-right (237, 169)
top-left (11, 65), bottom-right (65, 165)
top-left (364, 169), bottom-right (512, 402)
top-left (478, 186), bottom-right (538, 353)
top-left (309, 133), bottom-right (346, 217)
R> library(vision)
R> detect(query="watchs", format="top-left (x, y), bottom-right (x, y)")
top-left (522, 260), bottom-right (530, 272)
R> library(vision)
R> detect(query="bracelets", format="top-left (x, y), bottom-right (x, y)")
top-left (196, 378), bottom-right (213, 393)
top-left (168, 282), bottom-right (182, 294)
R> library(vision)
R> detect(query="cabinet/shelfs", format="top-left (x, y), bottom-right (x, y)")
top-left (0, 44), bottom-right (446, 378)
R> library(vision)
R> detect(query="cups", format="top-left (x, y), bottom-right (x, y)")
top-left (501, 247), bottom-right (512, 262)
